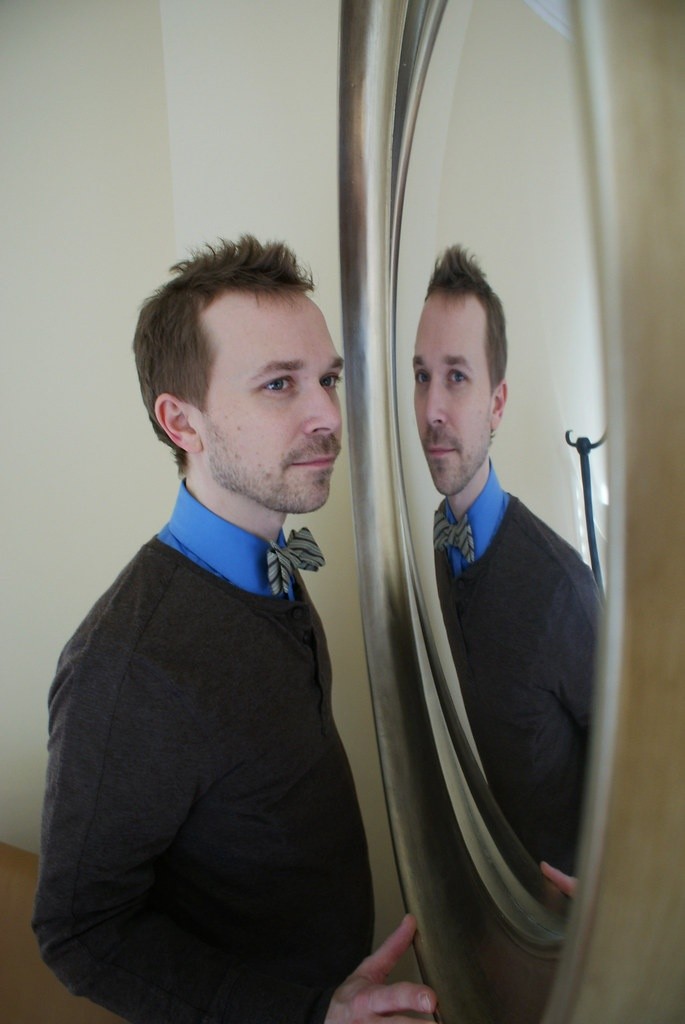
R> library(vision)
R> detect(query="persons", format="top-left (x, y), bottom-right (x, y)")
top-left (31, 235), bottom-right (437, 1024)
top-left (414, 247), bottom-right (601, 1016)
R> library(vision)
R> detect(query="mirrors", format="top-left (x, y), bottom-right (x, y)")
top-left (342, 0), bottom-right (685, 1024)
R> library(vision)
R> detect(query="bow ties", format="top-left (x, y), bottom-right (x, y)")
top-left (433, 509), bottom-right (475, 565)
top-left (267, 526), bottom-right (324, 597)
